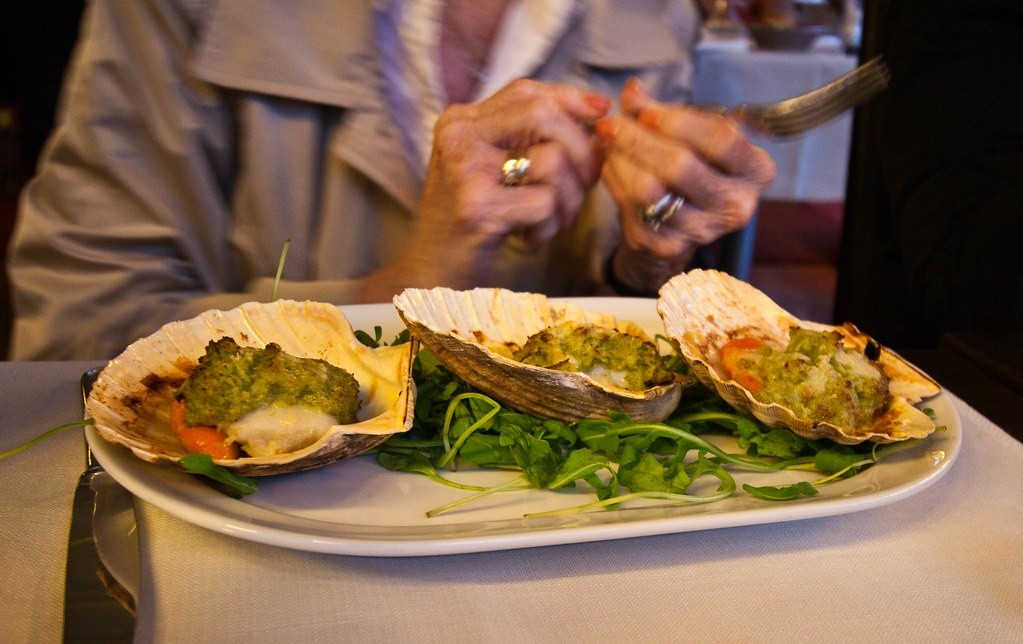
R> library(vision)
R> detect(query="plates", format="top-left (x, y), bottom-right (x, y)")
top-left (85, 296), bottom-right (963, 556)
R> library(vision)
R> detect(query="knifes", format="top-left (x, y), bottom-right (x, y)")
top-left (60, 366), bottom-right (140, 643)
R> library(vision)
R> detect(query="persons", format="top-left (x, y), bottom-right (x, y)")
top-left (9, 0), bottom-right (779, 360)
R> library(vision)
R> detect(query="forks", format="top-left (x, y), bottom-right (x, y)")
top-left (578, 53), bottom-right (892, 138)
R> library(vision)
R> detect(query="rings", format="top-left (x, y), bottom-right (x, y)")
top-left (501, 153), bottom-right (529, 184)
top-left (645, 191), bottom-right (685, 232)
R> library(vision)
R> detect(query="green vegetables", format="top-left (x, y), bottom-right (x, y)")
top-left (0, 237), bottom-right (948, 519)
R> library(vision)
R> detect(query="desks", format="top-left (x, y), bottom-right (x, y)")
top-left (1, 358), bottom-right (1023, 643)
